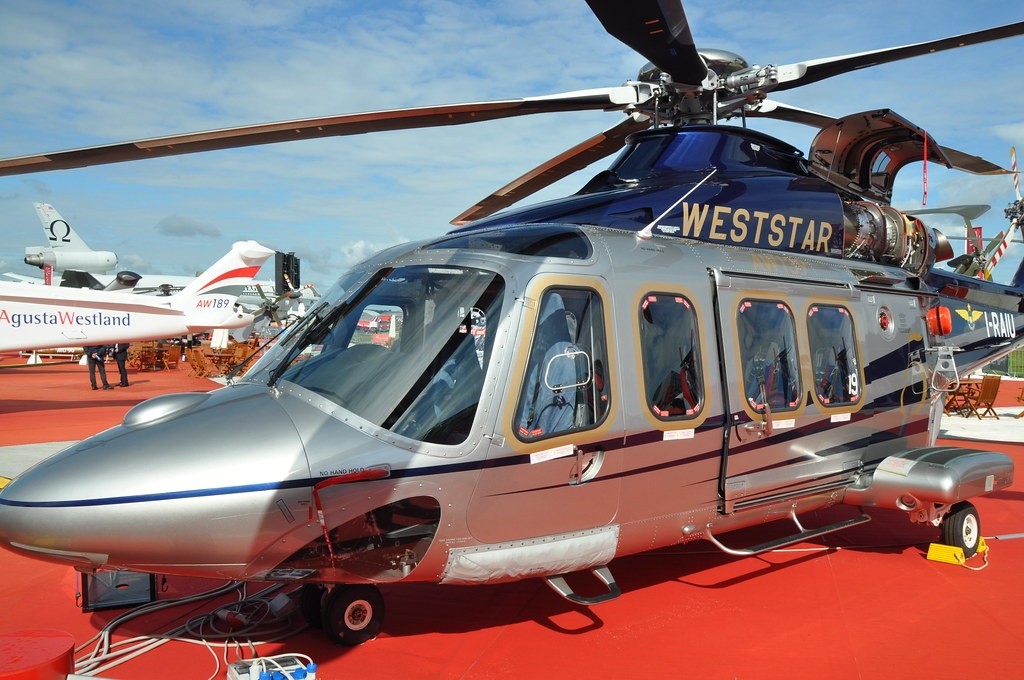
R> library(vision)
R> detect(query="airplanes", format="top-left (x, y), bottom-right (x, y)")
top-left (0, 201), bottom-right (330, 354)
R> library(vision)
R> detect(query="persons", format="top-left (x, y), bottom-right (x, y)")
top-left (83, 344), bottom-right (114, 389)
top-left (113, 343), bottom-right (130, 387)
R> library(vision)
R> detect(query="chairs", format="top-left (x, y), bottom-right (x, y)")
top-left (517, 290), bottom-right (581, 442)
top-left (967, 374), bottom-right (999, 421)
top-left (128, 334), bottom-right (259, 380)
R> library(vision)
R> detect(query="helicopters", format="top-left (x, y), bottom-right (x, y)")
top-left (0, 1), bottom-right (1024, 680)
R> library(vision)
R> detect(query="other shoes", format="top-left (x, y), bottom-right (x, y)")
top-left (115, 382), bottom-right (128, 386)
top-left (92, 386), bottom-right (97, 390)
top-left (103, 384), bottom-right (114, 389)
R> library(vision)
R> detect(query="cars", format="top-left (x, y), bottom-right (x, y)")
top-left (377, 321), bottom-right (390, 331)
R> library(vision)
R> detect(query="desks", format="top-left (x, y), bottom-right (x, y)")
top-left (946, 380), bottom-right (982, 417)
top-left (143, 348), bottom-right (168, 351)
top-left (205, 354), bottom-right (233, 377)
